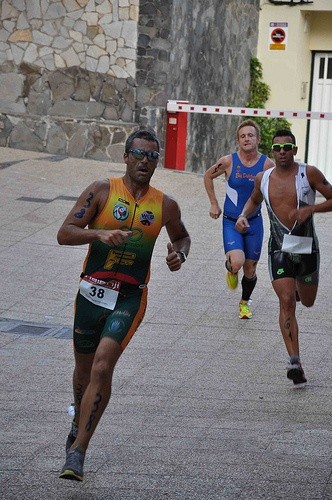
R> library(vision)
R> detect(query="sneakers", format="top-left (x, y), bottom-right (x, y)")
top-left (226, 271), bottom-right (238, 290)
top-left (59, 450), bottom-right (86, 482)
top-left (65, 418), bottom-right (79, 457)
top-left (238, 300), bottom-right (254, 318)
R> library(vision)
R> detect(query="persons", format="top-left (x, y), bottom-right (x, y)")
top-left (203, 119), bottom-right (275, 319)
top-left (235, 130), bottom-right (332, 385)
top-left (57, 130), bottom-right (191, 481)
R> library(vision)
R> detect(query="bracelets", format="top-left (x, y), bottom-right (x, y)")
top-left (238, 215), bottom-right (247, 218)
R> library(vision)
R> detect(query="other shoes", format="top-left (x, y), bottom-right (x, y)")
top-left (286, 360), bottom-right (307, 385)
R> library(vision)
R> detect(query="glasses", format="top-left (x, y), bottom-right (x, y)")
top-left (271, 142), bottom-right (297, 151)
top-left (127, 148), bottom-right (160, 162)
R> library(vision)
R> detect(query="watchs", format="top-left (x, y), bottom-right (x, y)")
top-left (176, 251), bottom-right (187, 263)
